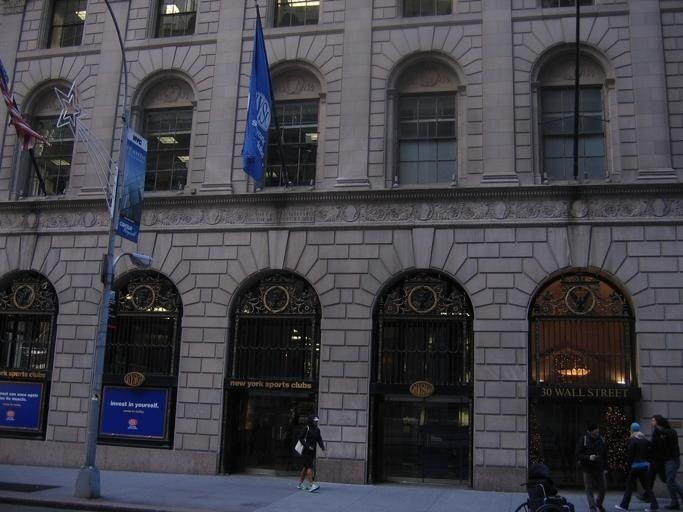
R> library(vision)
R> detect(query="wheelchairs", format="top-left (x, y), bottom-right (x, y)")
top-left (512, 481), bottom-right (571, 512)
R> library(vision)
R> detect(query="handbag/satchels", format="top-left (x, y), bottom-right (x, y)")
top-left (295, 441), bottom-right (305, 455)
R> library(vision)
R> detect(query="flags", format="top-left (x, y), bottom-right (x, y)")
top-left (2, 61), bottom-right (52, 148)
top-left (241, 5), bottom-right (272, 187)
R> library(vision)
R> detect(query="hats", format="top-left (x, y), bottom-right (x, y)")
top-left (630, 423), bottom-right (640, 432)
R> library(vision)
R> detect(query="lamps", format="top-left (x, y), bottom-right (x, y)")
top-left (554, 352), bottom-right (591, 377)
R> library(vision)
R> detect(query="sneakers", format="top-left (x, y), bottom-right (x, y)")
top-left (296, 481), bottom-right (305, 489)
top-left (590, 495), bottom-right (680, 512)
top-left (309, 483), bottom-right (320, 492)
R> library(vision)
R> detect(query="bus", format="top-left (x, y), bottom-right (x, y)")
top-left (374, 394), bottom-right (472, 432)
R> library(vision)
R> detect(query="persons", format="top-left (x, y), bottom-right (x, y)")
top-left (576, 422), bottom-right (609, 512)
top-left (655, 417), bottom-right (683, 510)
top-left (614, 421), bottom-right (662, 512)
top-left (296, 414), bottom-right (326, 491)
top-left (278, 1), bottom-right (298, 26)
top-left (636, 414), bottom-right (667, 502)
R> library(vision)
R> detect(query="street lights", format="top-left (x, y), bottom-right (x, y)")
top-left (67, 248), bottom-right (154, 500)
